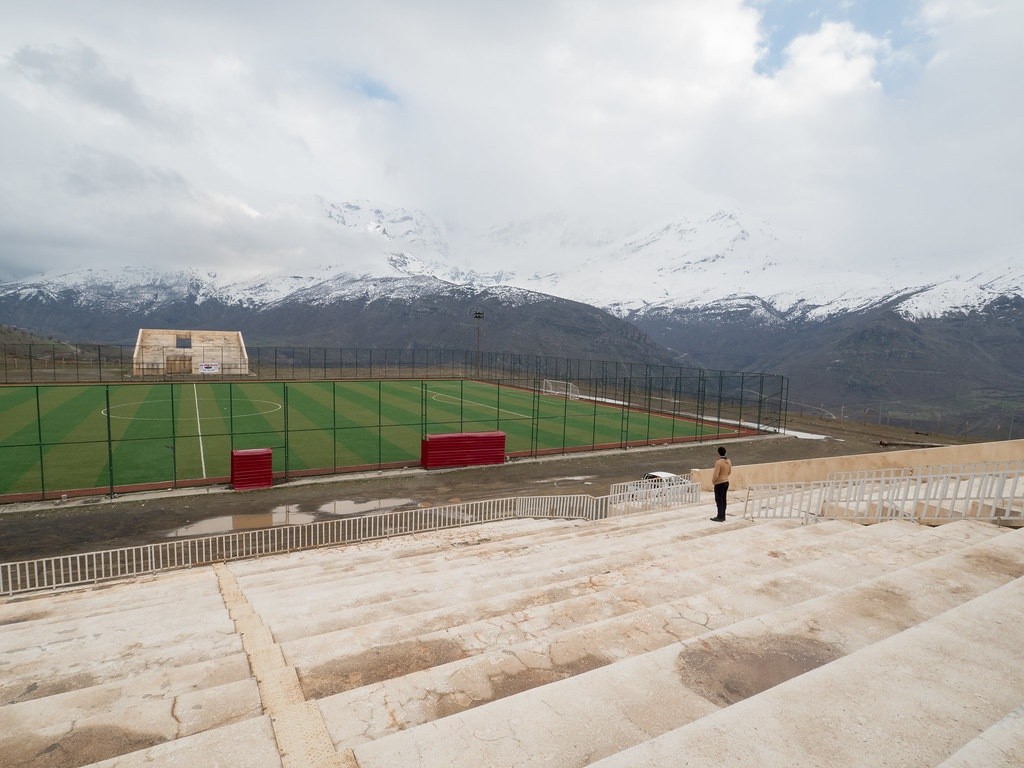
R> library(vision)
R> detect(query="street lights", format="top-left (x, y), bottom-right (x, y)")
top-left (474, 310), bottom-right (486, 375)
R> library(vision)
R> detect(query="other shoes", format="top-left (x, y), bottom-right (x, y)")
top-left (710, 517), bottom-right (723, 522)
top-left (723, 516), bottom-right (725, 521)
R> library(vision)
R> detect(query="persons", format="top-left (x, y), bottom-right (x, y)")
top-left (710, 447), bottom-right (732, 523)
